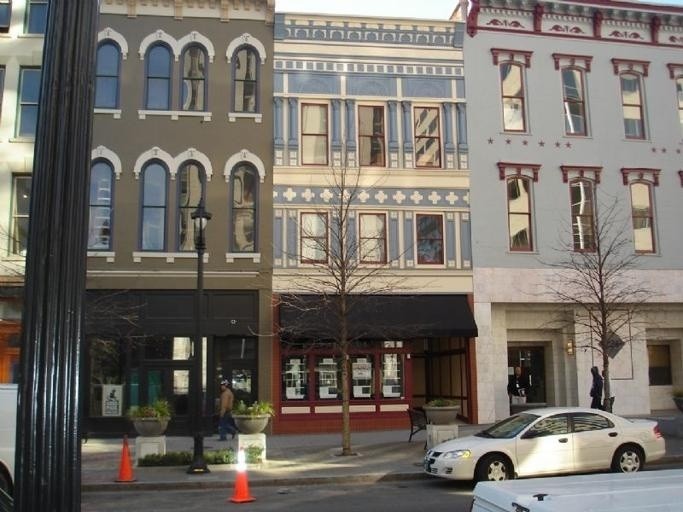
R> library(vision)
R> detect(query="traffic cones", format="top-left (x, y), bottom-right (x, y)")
top-left (114, 433), bottom-right (136, 482)
top-left (226, 446), bottom-right (256, 503)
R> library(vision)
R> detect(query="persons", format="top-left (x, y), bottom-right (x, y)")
top-left (590, 367), bottom-right (603, 410)
top-left (507, 367), bottom-right (529, 404)
top-left (602, 371), bottom-right (615, 413)
top-left (218, 380), bottom-right (236, 441)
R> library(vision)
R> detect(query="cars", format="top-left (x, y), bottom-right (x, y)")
top-left (0, 383), bottom-right (17, 500)
top-left (424, 406), bottom-right (667, 488)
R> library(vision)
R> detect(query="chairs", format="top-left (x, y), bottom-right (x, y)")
top-left (407, 409), bottom-right (431, 449)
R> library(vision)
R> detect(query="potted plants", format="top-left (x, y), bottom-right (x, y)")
top-left (231, 399), bottom-right (276, 433)
top-left (127, 398), bottom-right (173, 434)
top-left (423, 398), bottom-right (460, 424)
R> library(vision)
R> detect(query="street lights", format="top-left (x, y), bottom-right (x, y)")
top-left (186, 196), bottom-right (213, 477)
